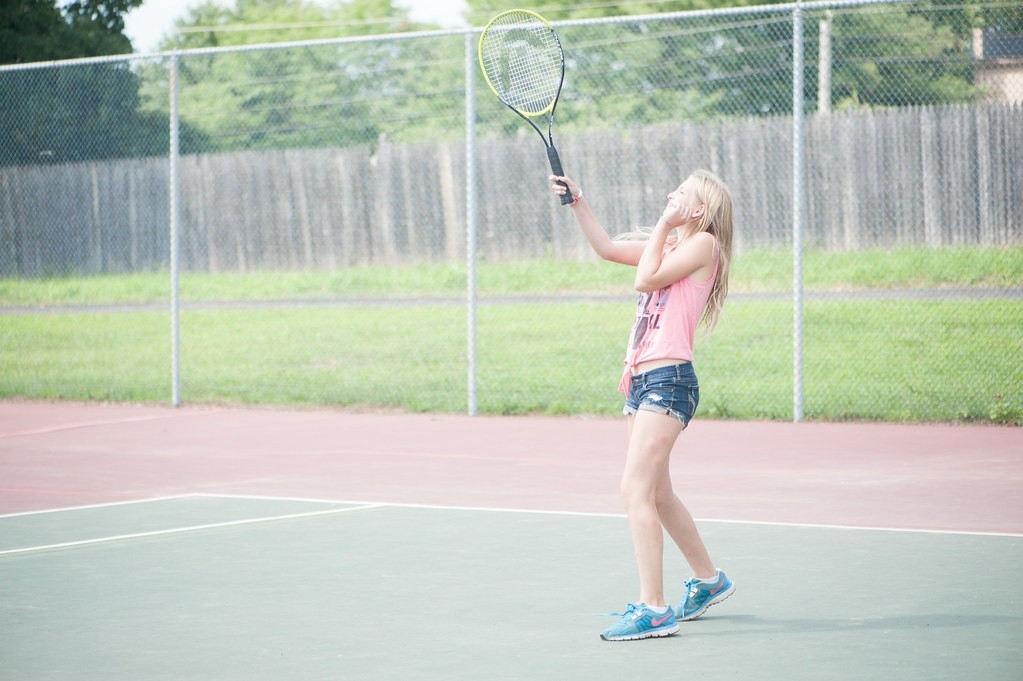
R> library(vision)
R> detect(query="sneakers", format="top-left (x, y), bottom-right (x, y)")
top-left (674, 568), bottom-right (736, 622)
top-left (592, 603), bottom-right (680, 642)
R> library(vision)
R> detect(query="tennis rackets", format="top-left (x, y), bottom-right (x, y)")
top-left (478, 8), bottom-right (575, 206)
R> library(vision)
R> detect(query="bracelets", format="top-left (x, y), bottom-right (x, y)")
top-left (567, 188), bottom-right (583, 207)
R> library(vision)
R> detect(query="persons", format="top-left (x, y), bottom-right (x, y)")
top-left (547, 170), bottom-right (734, 642)
